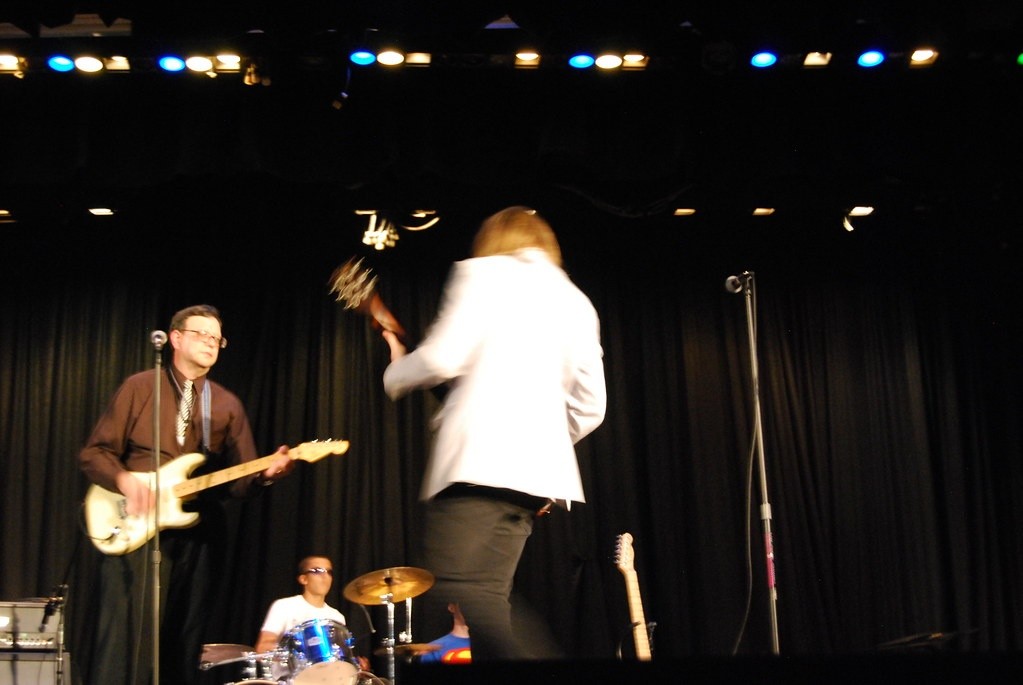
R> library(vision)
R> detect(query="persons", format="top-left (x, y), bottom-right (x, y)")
top-left (254, 549), bottom-right (372, 674)
top-left (79, 302), bottom-right (295, 684)
top-left (382, 205), bottom-right (607, 658)
top-left (416, 597), bottom-right (472, 665)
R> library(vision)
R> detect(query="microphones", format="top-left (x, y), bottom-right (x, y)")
top-left (151, 330), bottom-right (167, 349)
top-left (42, 588), bottom-right (58, 627)
top-left (725, 272), bottom-right (754, 293)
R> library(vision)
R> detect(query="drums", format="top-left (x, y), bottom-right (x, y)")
top-left (228, 618), bottom-right (395, 685)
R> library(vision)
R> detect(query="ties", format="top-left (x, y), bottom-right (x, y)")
top-left (175, 379), bottom-right (194, 446)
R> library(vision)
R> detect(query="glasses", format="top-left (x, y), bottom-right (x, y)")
top-left (177, 329), bottom-right (226, 348)
top-left (301, 568), bottom-right (333, 576)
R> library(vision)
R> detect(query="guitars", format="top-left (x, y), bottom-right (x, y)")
top-left (81, 437), bottom-right (351, 557)
top-left (613, 531), bottom-right (654, 663)
top-left (326, 252), bottom-right (452, 404)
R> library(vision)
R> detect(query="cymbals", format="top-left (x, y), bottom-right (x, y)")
top-left (374, 643), bottom-right (442, 657)
top-left (198, 642), bottom-right (258, 673)
top-left (341, 565), bottom-right (436, 607)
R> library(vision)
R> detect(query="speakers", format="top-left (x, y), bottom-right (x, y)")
top-left (0, 651), bottom-right (71, 685)
